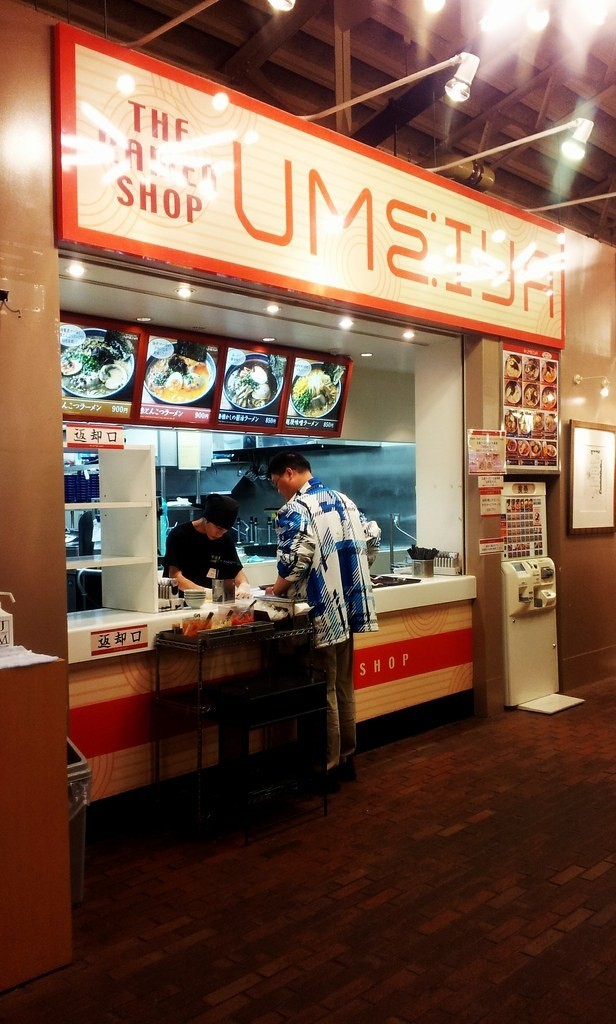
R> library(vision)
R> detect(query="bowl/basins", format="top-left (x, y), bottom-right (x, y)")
top-left (222, 352), bottom-right (283, 413)
top-left (290, 361), bottom-right (342, 418)
top-left (61, 328), bottom-right (135, 400)
top-left (504, 355), bottom-right (557, 435)
top-left (144, 341), bottom-right (217, 406)
top-left (184, 589), bottom-right (206, 609)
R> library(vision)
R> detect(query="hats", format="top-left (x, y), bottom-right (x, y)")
top-left (201, 494), bottom-right (240, 530)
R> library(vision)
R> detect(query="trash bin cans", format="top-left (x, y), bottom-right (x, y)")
top-left (66, 732), bottom-right (93, 912)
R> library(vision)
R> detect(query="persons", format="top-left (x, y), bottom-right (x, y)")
top-left (163, 492), bottom-right (251, 602)
top-left (262, 448), bottom-right (382, 797)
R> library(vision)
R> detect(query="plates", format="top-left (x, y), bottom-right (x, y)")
top-left (506, 439), bottom-right (557, 458)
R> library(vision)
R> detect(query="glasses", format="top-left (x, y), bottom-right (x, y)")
top-left (272, 467), bottom-right (297, 487)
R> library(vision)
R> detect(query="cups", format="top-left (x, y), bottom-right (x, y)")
top-left (411, 559), bottom-right (433, 578)
top-left (212, 578), bottom-right (235, 604)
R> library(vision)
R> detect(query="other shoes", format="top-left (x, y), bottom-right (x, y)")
top-left (282, 767), bottom-right (344, 800)
top-left (337, 756), bottom-right (356, 782)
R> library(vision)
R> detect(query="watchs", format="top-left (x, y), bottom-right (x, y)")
top-left (270, 586), bottom-right (276, 597)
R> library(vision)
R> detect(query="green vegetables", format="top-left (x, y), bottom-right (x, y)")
top-left (292, 388), bottom-right (330, 412)
top-left (226, 374), bottom-right (260, 395)
top-left (152, 368), bottom-right (193, 386)
top-left (64, 345), bottom-right (111, 377)
